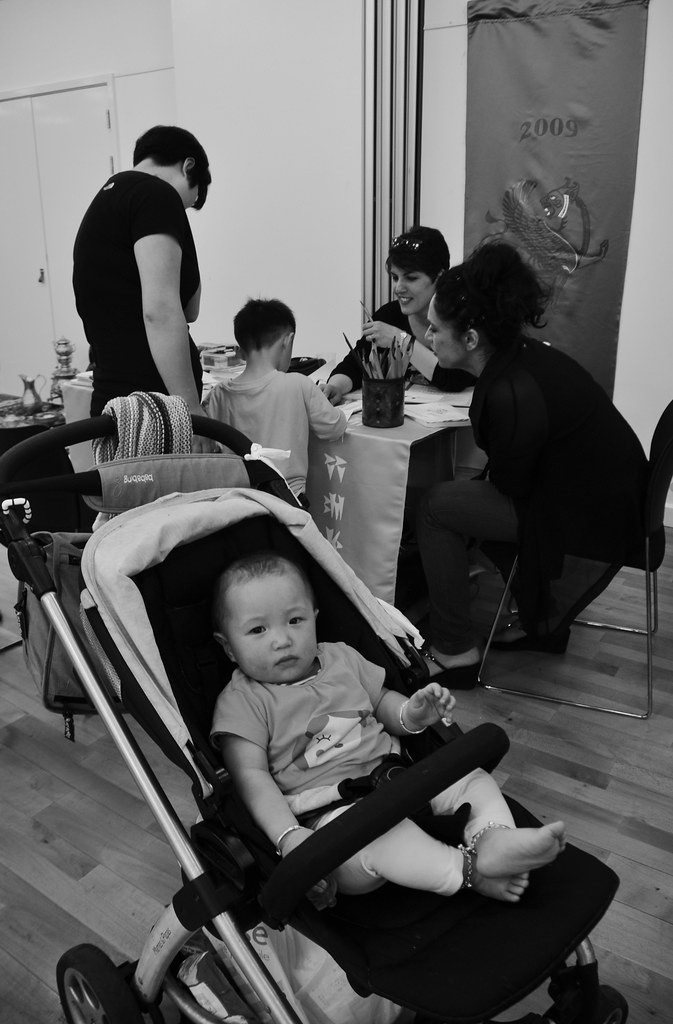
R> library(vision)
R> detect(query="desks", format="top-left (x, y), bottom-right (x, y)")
top-left (62, 371), bottom-right (471, 605)
top-left (0, 393), bottom-right (64, 458)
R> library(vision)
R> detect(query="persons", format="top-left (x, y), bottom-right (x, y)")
top-left (317, 226), bottom-right (478, 406)
top-left (73, 126), bottom-right (212, 453)
top-left (211, 556), bottom-right (566, 903)
top-left (419, 244), bottom-right (648, 696)
top-left (202, 299), bottom-right (346, 511)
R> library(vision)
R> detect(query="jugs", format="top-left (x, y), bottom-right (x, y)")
top-left (17, 373), bottom-right (45, 412)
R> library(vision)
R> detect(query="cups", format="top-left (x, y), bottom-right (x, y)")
top-left (363, 377), bottom-right (405, 428)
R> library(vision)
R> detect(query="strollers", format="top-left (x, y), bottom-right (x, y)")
top-left (0, 408), bottom-right (631, 1024)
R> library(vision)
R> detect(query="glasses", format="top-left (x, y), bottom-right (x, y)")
top-left (388, 236), bottom-right (424, 251)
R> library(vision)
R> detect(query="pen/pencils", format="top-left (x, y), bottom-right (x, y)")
top-left (342, 332), bottom-right (416, 379)
top-left (360, 301), bottom-right (375, 323)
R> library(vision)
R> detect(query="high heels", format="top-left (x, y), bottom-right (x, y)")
top-left (422, 645), bottom-right (483, 690)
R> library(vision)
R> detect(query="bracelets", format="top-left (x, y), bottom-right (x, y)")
top-left (276, 825), bottom-right (303, 855)
top-left (399, 700), bottom-right (427, 734)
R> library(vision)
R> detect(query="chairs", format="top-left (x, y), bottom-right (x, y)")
top-left (0, 424), bottom-right (87, 601)
top-left (476, 400), bottom-right (673, 719)
